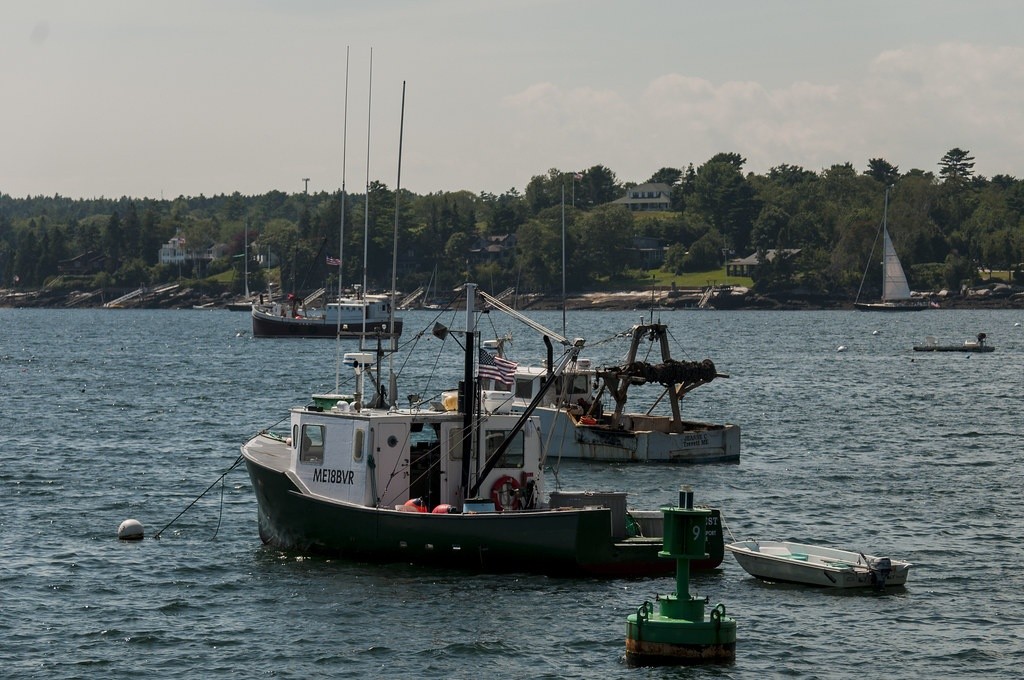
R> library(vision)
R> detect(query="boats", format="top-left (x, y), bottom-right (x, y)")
top-left (726, 539), bottom-right (912, 588)
top-left (912, 334), bottom-right (996, 352)
top-left (245, 242), bottom-right (405, 339)
top-left (192, 302), bottom-right (230, 311)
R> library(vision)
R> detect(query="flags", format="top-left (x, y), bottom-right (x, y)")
top-left (326, 257), bottom-right (340, 265)
top-left (479, 349), bottom-right (517, 384)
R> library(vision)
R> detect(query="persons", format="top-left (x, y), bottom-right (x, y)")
top-left (293, 305), bottom-right (299, 318)
top-left (962, 282), bottom-right (967, 296)
top-left (281, 310), bottom-right (286, 317)
top-left (577, 398), bottom-right (589, 413)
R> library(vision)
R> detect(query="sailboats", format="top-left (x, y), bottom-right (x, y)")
top-left (226, 221), bottom-right (254, 312)
top-left (853, 188), bottom-right (932, 311)
top-left (239, 40), bottom-right (725, 578)
top-left (648, 283), bottom-right (676, 311)
top-left (432, 185), bottom-right (742, 466)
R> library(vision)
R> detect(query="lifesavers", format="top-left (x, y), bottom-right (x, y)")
top-left (491, 473), bottom-right (522, 511)
